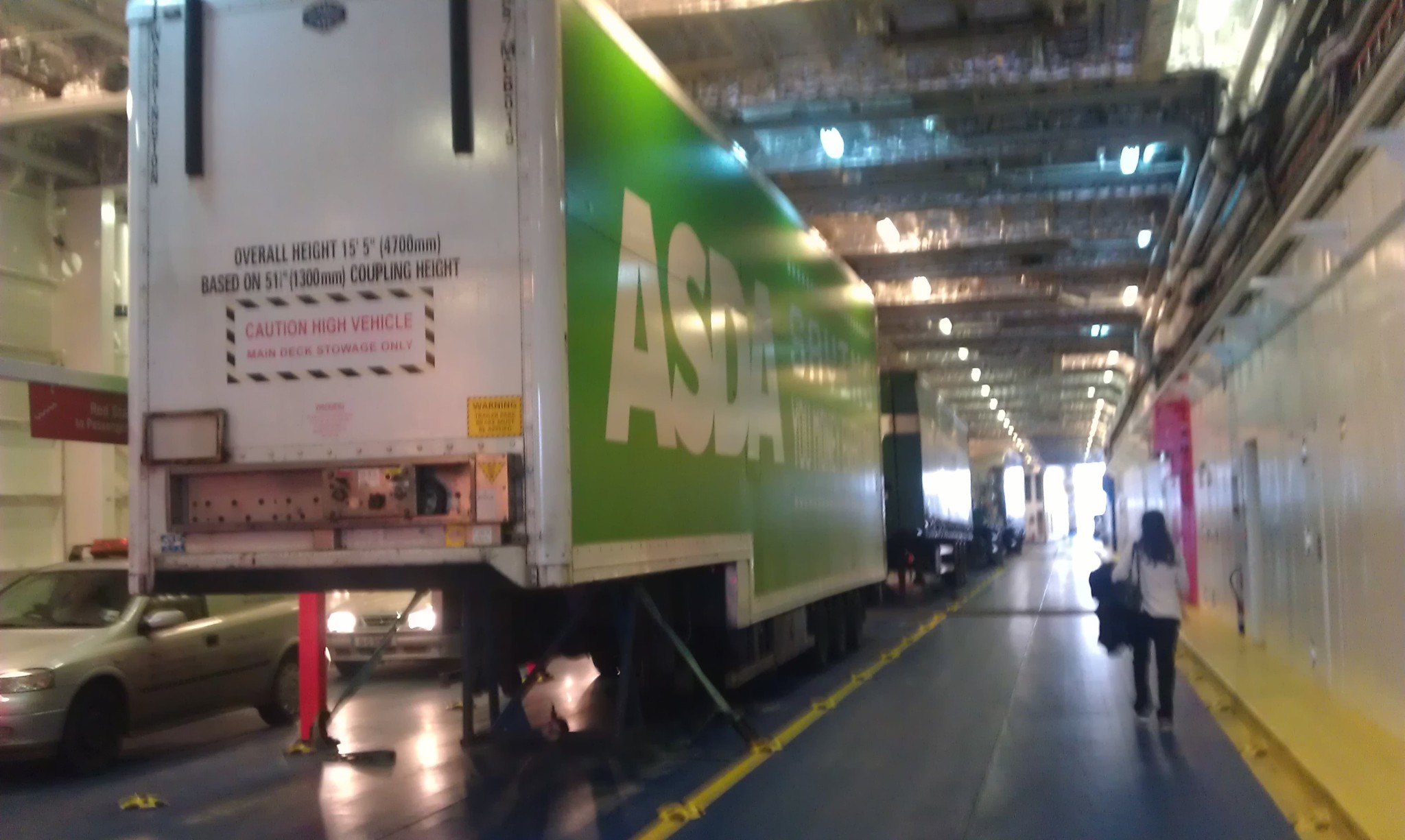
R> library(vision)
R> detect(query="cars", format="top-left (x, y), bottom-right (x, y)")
top-left (324, 586), bottom-right (460, 680)
top-left (0, 537), bottom-right (300, 781)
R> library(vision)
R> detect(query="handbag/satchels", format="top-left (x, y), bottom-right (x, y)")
top-left (1112, 543), bottom-right (1142, 618)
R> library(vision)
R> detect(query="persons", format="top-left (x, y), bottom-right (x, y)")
top-left (1111, 510), bottom-right (1184, 725)
